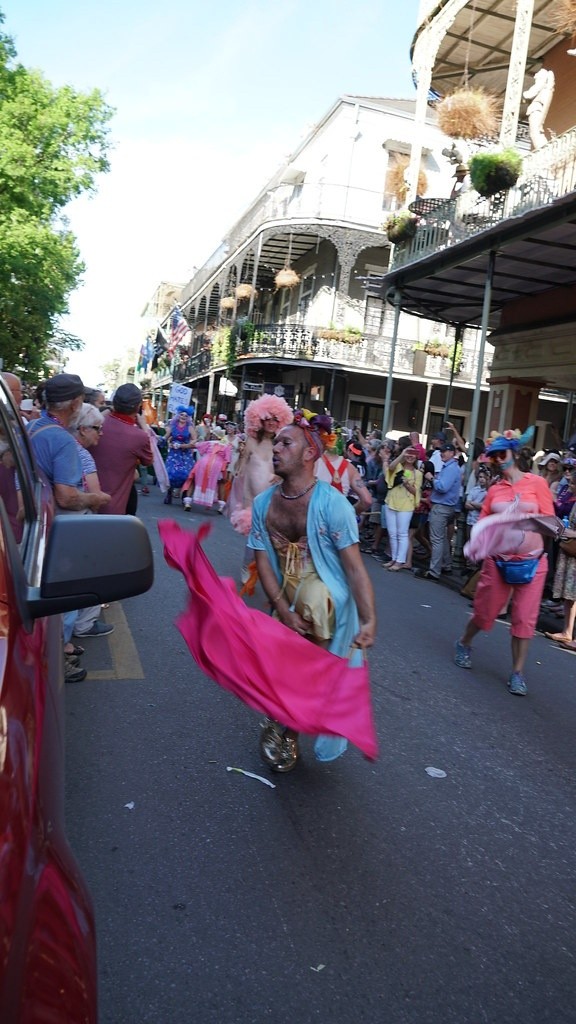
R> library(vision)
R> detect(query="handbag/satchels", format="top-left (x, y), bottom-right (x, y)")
top-left (494, 550), bottom-right (544, 583)
top-left (559, 535), bottom-right (576, 558)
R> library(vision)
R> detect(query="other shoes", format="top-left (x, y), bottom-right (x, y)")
top-left (559, 640), bottom-right (576, 651)
top-left (358, 533), bottom-right (392, 563)
top-left (259, 725), bottom-right (285, 766)
top-left (496, 613), bottom-right (507, 619)
top-left (461, 588), bottom-right (475, 601)
top-left (240, 570), bottom-right (252, 585)
top-left (442, 568), bottom-right (452, 575)
top-left (414, 570), bottom-right (439, 582)
top-left (136, 474), bottom-right (227, 513)
top-left (541, 597), bottom-right (565, 618)
top-left (388, 565), bottom-right (402, 572)
top-left (382, 563), bottom-right (392, 568)
top-left (273, 737), bottom-right (297, 770)
top-left (545, 631), bottom-right (572, 643)
top-left (63, 619), bottom-right (115, 682)
top-left (402, 563), bottom-right (412, 570)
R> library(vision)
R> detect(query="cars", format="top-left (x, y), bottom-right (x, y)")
top-left (0, 372), bottom-right (155, 1024)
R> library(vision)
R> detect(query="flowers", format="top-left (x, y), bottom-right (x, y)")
top-left (294, 407), bottom-right (337, 449)
top-left (486, 428), bottom-right (523, 444)
top-left (382, 213), bottom-right (416, 240)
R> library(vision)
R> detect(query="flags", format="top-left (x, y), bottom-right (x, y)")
top-left (137, 327), bottom-right (169, 374)
top-left (169, 305), bottom-right (191, 359)
top-left (160, 518), bottom-right (379, 762)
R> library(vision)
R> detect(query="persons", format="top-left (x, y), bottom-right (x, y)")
top-left (521, 69), bottom-right (555, 149)
top-left (0, 412), bottom-right (37, 549)
top-left (247, 407), bottom-right (377, 773)
top-left (1, 371), bottom-right (157, 682)
top-left (316, 421), bottom-right (576, 648)
top-left (453, 425), bottom-right (555, 694)
top-left (164, 395), bottom-right (292, 583)
top-left (451, 165), bottom-right (472, 197)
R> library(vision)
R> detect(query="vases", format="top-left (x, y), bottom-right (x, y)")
top-left (388, 218), bottom-right (412, 242)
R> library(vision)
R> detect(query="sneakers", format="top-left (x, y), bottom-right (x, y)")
top-left (506, 672), bottom-right (527, 695)
top-left (454, 638), bottom-right (472, 668)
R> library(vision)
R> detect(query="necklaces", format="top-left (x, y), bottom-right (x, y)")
top-left (279, 476), bottom-right (319, 499)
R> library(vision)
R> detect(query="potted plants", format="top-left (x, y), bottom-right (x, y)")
top-left (469, 150), bottom-right (523, 197)
top-left (409, 340), bottom-right (448, 378)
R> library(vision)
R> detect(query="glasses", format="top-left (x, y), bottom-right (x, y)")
top-left (490, 449), bottom-right (512, 463)
top-left (561, 465), bottom-right (575, 473)
top-left (74, 423), bottom-right (104, 434)
top-left (549, 459), bottom-right (559, 464)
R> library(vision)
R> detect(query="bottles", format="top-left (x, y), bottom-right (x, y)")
top-left (560, 516), bottom-right (569, 539)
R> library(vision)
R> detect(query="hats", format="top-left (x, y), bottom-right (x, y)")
top-left (563, 457), bottom-right (576, 465)
top-left (43, 372), bottom-right (141, 411)
top-left (539, 452), bottom-right (560, 465)
top-left (435, 444), bottom-right (455, 452)
